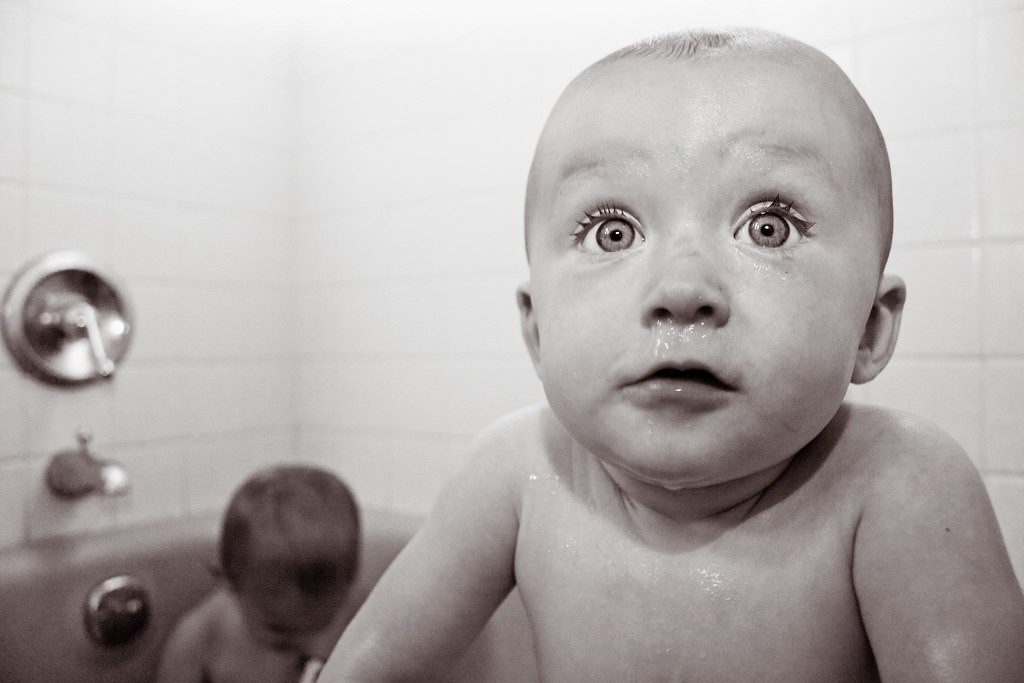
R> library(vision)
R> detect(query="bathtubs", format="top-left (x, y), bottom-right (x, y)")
top-left (0, 507), bottom-right (539, 681)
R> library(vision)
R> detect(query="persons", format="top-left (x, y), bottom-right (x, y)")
top-left (158, 465), bottom-right (363, 683)
top-left (313, 27), bottom-right (1024, 683)
